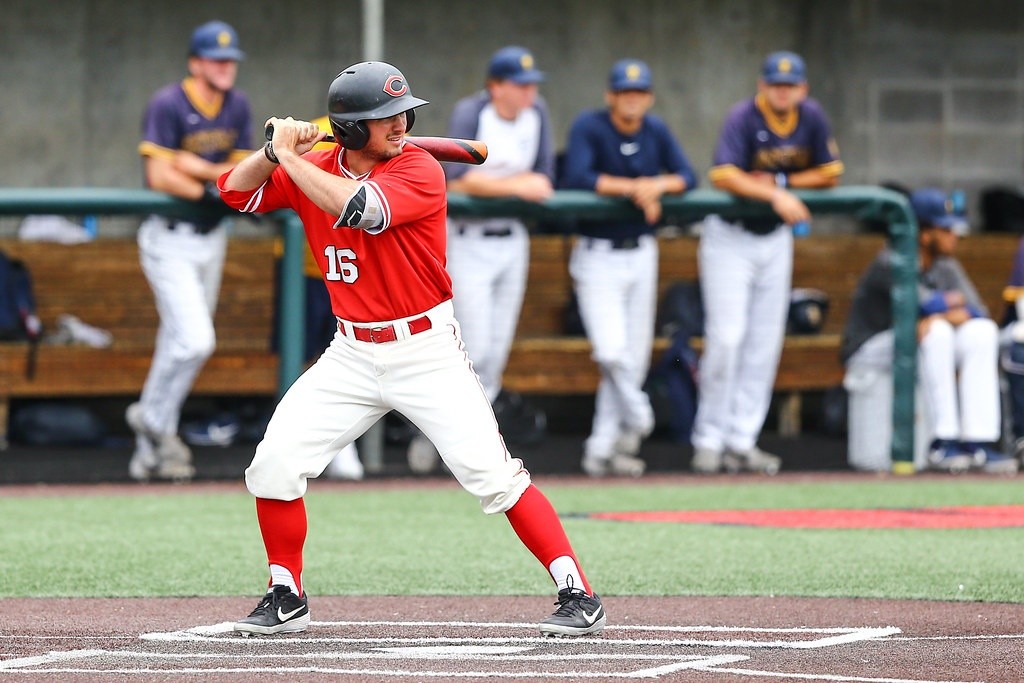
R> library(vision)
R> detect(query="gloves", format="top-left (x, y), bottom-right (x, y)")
top-left (195, 192), bottom-right (224, 233)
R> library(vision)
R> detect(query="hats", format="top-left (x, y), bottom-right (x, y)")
top-left (609, 59), bottom-right (653, 91)
top-left (911, 189), bottom-right (964, 230)
top-left (764, 50), bottom-right (806, 84)
top-left (189, 20), bottom-right (243, 60)
top-left (489, 46), bottom-right (544, 85)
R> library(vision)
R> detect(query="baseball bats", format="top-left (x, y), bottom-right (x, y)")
top-left (265, 122), bottom-right (488, 167)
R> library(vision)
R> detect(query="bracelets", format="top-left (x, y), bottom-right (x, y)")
top-left (773, 171), bottom-right (790, 188)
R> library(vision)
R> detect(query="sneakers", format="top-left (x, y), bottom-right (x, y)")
top-left (234, 585), bottom-right (311, 637)
top-left (539, 574), bottom-right (606, 636)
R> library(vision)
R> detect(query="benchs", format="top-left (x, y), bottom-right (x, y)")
top-left (0, 232), bottom-right (1024, 447)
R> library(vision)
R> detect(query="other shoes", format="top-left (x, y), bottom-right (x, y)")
top-left (687, 446), bottom-right (782, 475)
top-left (930, 442), bottom-right (971, 470)
top-left (408, 436), bottom-right (441, 475)
top-left (582, 449), bottom-right (647, 477)
top-left (126, 412), bottom-right (195, 480)
top-left (976, 447), bottom-right (1020, 474)
top-left (328, 444), bottom-right (365, 483)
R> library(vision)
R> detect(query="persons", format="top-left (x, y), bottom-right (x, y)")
top-left (559, 56), bottom-right (700, 480)
top-left (689, 49), bottom-right (845, 475)
top-left (838, 186), bottom-right (1020, 474)
top-left (267, 115), bottom-right (365, 480)
top-left (999, 236), bottom-right (1024, 464)
top-left (123, 20), bottom-right (252, 480)
top-left (406, 44), bottom-right (556, 476)
top-left (215, 60), bottom-right (608, 637)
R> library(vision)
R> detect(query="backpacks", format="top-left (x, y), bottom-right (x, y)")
top-left (0, 252), bottom-right (43, 343)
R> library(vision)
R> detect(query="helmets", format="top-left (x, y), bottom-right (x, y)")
top-left (327, 61), bottom-right (429, 150)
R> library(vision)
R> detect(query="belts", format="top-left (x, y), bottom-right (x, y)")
top-left (458, 227), bottom-right (512, 238)
top-left (587, 237), bottom-right (638, 251)
top-left (338, 317), bottom-right (431, 343)
top-left (744, 220), bottom-right (778, 234)
